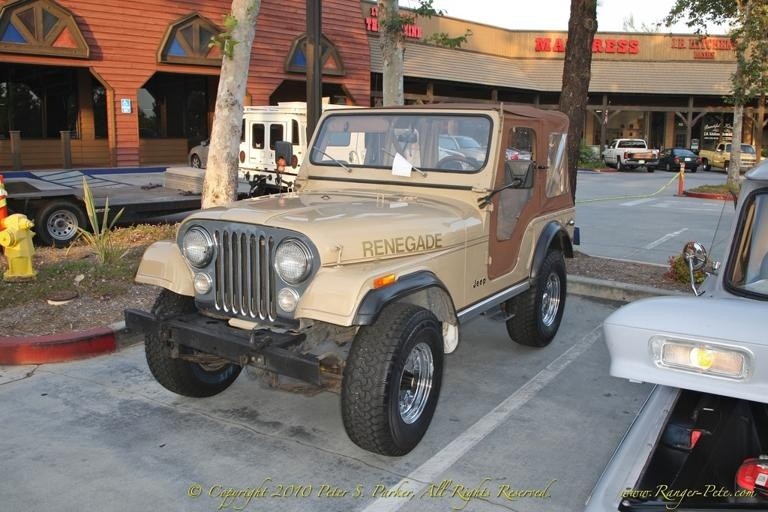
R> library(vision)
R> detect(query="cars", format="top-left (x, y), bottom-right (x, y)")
top-left (503, 143), bottom-right (532, 165)
top-left (439, 132), bottom-right (499, 172)
top-left (583, 158), bottom-right (767, 512)
top-left (657, 148), bottom-right (702, 173)
top-left (188, 136), bottom-right (210, 169)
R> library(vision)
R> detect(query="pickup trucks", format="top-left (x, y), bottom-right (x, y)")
top-left (698, 141), bottom-right (756, 175)
top-left (602, 138), bottom-right (660, 174)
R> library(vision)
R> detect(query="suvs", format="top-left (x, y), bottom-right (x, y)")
top-left (135, 99), bottom-right (582, 458)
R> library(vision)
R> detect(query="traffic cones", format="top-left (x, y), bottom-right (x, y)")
top-left (0, 175), bottom-right (10, 231)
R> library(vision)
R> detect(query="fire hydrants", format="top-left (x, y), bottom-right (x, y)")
top-left (0, 213), bottom-right (40, 282)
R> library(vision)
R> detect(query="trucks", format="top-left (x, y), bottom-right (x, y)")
top-left (2, 95), bottom-right (422, 248)
